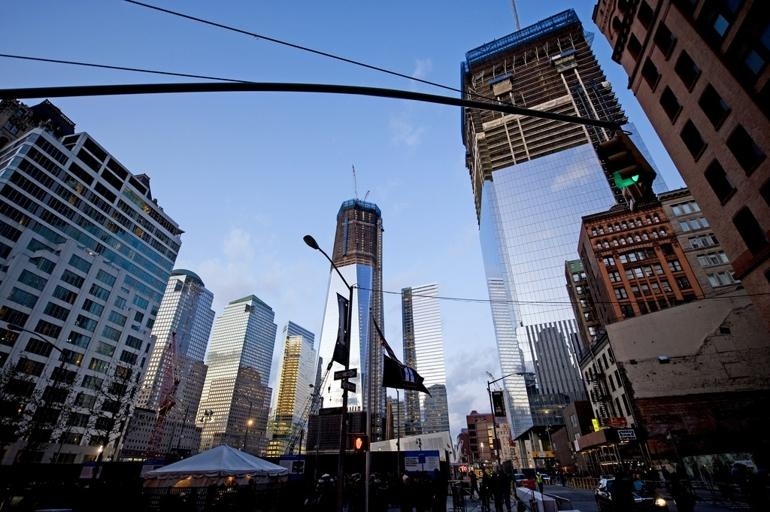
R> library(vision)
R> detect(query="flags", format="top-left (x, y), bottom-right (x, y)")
top-left (381, 354), bottom-right (433, 397)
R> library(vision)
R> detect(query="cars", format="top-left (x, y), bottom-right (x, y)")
top-left (541, 474), bottom-right (551, 480)
top-left (514, 473), bottom-right (528, 483)
top-left (595, 478), bottom-right (696, 512)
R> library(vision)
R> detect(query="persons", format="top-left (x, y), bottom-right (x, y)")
top-left (536, 472), bottom-right (544, 493)
top-left (306, 468), bottom-right (512, 511)
top-left (609, 456), bottom-right (769, 511)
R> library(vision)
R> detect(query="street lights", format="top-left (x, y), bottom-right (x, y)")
top-left (238, 390), bottom-right (252, 449)
top-left (487, 371), bottom-right (535, 467)
top-left (303, 236), bottom-right (352, 511)
top-left (1, 324), bottom-right (68, 512)
top-left (170, 393), bottom-right (189, 447)
top-left (308, 384), bottom-right (324, 470)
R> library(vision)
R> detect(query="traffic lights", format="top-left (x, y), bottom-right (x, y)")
top-left (353, 434), bottom-right (369, 452)
top-left (597, 132), bottom-right (659, 199)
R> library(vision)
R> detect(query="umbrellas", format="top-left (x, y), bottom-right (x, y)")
top-left (145, 444), bottom-right (288, 478)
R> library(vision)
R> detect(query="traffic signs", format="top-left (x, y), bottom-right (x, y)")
top-left (334, 369), bottom-right (357, 379)
top-left (341, 380), bottom-right (356, 393)
